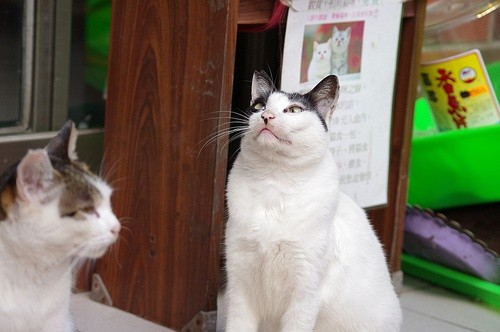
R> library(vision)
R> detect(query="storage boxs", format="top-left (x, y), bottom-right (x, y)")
top-left (406, 61), bottom-right (499, 210)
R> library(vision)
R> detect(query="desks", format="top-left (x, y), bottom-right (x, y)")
top-left (92, 0), bottom-right (427, 332)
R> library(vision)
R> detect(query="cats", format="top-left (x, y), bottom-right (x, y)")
top-left (307, 26), bottom-right (352, 82)
top-left (191, 61), bottom-right (403, 332)
top-left (0, 119), bottom-right (136, 332)
